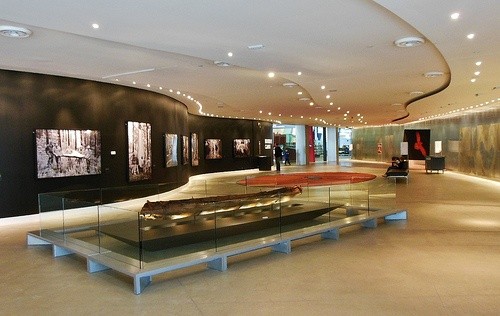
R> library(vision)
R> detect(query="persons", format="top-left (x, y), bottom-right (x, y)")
top-left (383, 155), bottom-right (406, 178)
top-left (275, 143), bottom-right (291, 172)
top-left (129, 153), bottom-right (149, 175)
top-left (45, 140), bottom-right (93, 173)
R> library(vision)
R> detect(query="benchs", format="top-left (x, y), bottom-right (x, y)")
top-left (425, 156), bottom-right (446, 174)
top-left (385, 161), bottom-right (409, 185)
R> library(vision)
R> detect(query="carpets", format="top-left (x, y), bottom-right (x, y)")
top-left (238, 173), bottom-right (377, 187)
